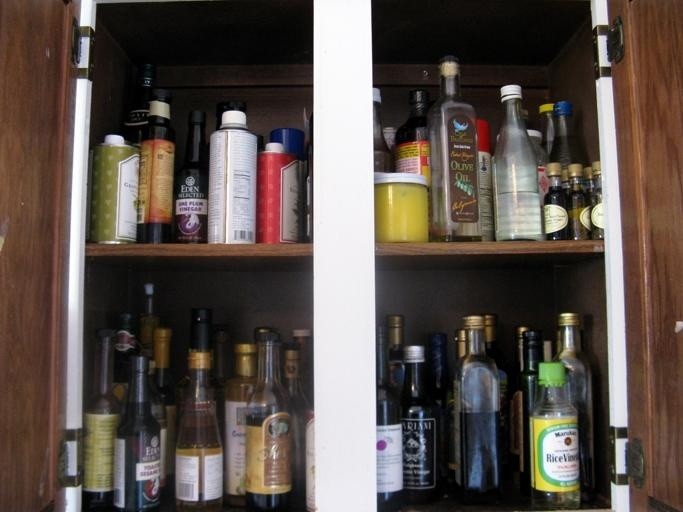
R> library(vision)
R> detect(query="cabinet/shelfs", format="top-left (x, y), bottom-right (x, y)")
top-left (84, 222), bottom-right (611, 512)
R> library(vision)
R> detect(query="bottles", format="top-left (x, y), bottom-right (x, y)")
top-left (90, 58), bottom-right (312, 245)
top-left (373, 54), bottom-right (604, 243)
top-left (378, 300), bottom-right (596, 512)
top-left (81, 281), bottom-right (313, 511)
